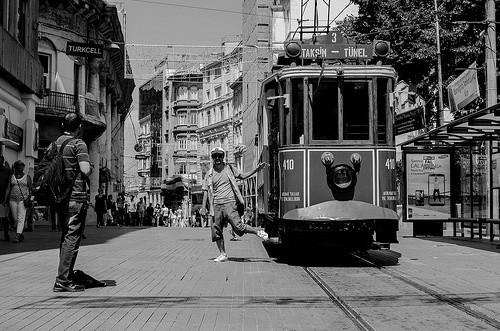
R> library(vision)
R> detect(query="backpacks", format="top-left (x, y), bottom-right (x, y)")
top-left (31, 133), bottom-right (76, 209)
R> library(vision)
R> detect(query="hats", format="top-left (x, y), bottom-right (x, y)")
top-left (211, 146), bottom-right (225, 157)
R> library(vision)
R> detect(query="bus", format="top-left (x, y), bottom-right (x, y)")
top-left (256, 0), bottom-right (403, 265)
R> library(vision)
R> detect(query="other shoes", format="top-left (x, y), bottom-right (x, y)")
top-left (52, 222), bottom-right (60, 232)
top-left (4, 233), bottom-right (10, 240)
top-left (12, 232), bottom-right (24, 243)
top-left (96, 225), bottom-right (101, 228)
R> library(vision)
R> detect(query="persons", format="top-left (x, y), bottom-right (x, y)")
top-left (419, 190), bottom-right (424, 205)
top-left (245, 201), bottom-right (256, 227)
top-left (226, 220), bottom-right (238, 241)
top-left (425, 176), bottom-right (447, 202)
top-left (94, 188), bottom-right (209, 228)
top-left (45, 113), bottom-right (95, 292)
top-left (0, 155), bottom-right (32, 242)
top-left (200, 147), bottom-right (269, 262)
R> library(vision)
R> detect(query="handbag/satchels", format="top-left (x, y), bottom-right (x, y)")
top-left (235, 199), bottom-right (245, 217)
top-left (24, 195), bottom-right (32, 208)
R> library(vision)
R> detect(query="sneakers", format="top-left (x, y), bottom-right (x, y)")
top-left (53, 272), bottom-right (93, 292)
top-left (256, 227), bottom-right (268, 242)
top-left (214, 253), bottom-right (228, 262)
